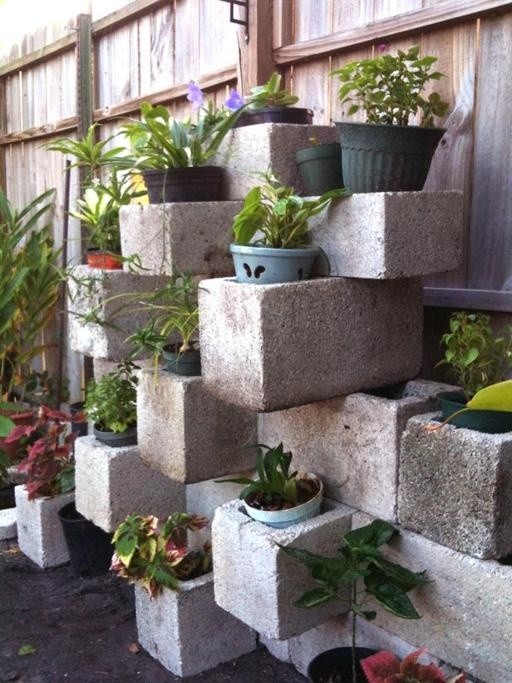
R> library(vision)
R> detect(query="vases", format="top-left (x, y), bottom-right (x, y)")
top-left (140, 159), bottom-right (229, 208)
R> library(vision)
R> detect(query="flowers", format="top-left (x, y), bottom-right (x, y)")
top-left (118, 80), bottom-right (247, 167)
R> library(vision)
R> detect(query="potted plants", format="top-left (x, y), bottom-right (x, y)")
top-left (276, 513), bottom-right (431, 683)
top-left (229, 162), bottom-right (327, 288)
top-left (225, 69), bottom-right (317, 125)
top-left (213, 440), bottom-right (327, 530)
top-left (432, 307), bottom-right (512, 432)
top-left (67, 184), bottom-right (127, 271)
top-left (328, 42), bottom-right (451, 195)
top-left (80, 269), bottom-right (222, 378)
top-left (83, 360), bottom-right (140, 450)
top-left (294, 135), bottom-right (345, 196)
